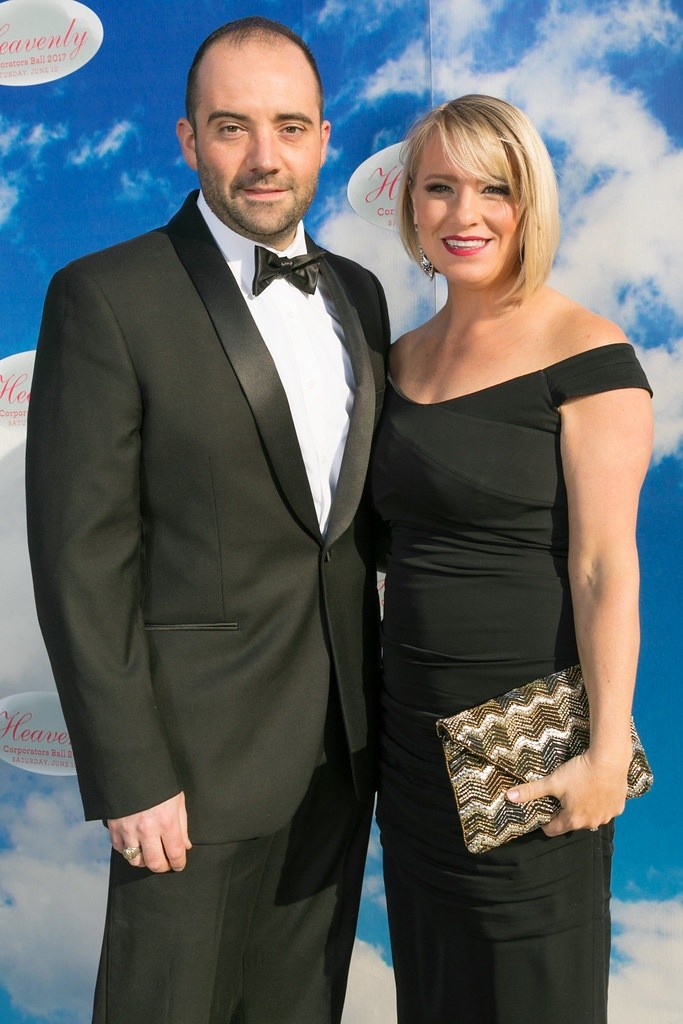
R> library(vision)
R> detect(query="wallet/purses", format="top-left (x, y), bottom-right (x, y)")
top-left (437, 662), bottom-right (654, 859)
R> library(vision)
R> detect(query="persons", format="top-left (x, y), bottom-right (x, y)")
top-left (372, 94), bottom-right (653, 1024)
top-left (24, 15), bottom-right (392, 1024)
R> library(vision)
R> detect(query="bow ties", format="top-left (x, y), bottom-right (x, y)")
top-left (251, 246), bottom-right (326, 293)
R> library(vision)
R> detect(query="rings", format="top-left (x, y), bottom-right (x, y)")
top-left (120, 846), bottom-right (142, 860)
